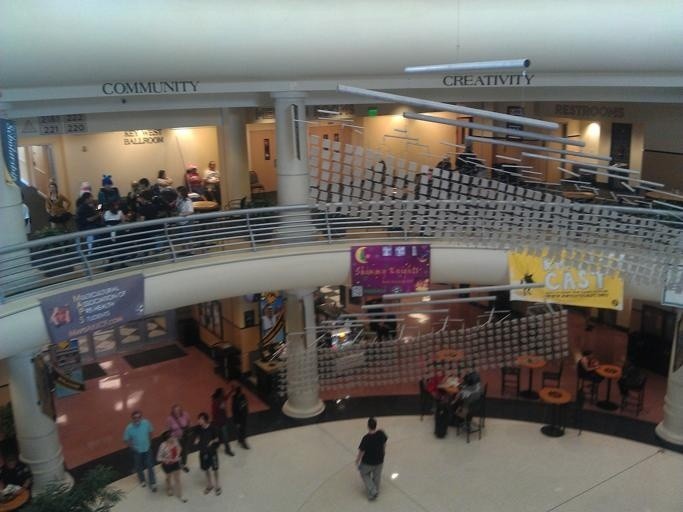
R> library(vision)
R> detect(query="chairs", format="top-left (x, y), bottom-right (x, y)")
top-left (421, 347), bottom-right (648, 443)
top-left (58, 170), bottom-right (265, 265)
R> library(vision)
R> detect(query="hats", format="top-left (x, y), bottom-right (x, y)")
top-left (81, 184), bottom-right (91, 193)
top-left (102, 174), bottom-right (112, 186)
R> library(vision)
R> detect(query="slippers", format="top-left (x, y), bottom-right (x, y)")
top-left (204, 486), bottom-right (214, 493)
top-left (216, 488), bottom-right (222, 495)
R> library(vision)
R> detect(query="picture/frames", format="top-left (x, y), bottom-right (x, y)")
top-left (506, 105), bottom-right (524, 141)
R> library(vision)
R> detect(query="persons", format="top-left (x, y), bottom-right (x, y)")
top-left (450, 371), bottom-right (486, 423)
top-left (616, 354), bottom-right (644, 398)
top-left (20, 159), bottom-right (220, 261)
top-left (455, 143), bottom-right (481, 172)
top-left (427, 366), bottom-right (446, 402)
top-left (577, 351), bottom-right (603, 394)
top-left (0, 455), bottom-right (33, 493)
top-left (122, 382), bottom-right (249, 504)
top-left (355, 414), bottom-right (388, 503)
top-left (258, 301), bottom-right (276, 331)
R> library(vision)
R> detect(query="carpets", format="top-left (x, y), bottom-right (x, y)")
top-left (122, 344), bottom-right (188, 369)
top-left (80, 364), bottom-right (108, 382)
